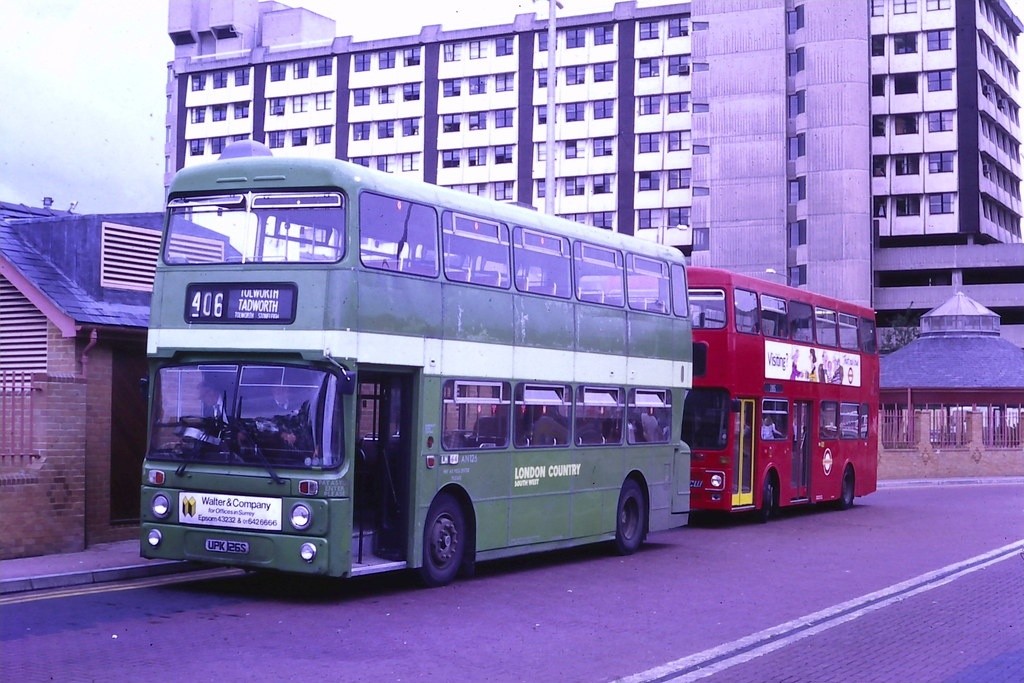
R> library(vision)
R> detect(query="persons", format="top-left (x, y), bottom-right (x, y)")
top-left (761, 415), bottom-right (782, 439)
top-left (190, 376), bottom-right (304, 448)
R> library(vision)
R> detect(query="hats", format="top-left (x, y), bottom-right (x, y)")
top-left (196, 381), bottom-right (222, 391)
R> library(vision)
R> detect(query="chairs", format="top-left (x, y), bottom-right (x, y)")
top-left (445, 411), bottom-right (667, 451)
top-left (361, 259), bottom-right (670, 315)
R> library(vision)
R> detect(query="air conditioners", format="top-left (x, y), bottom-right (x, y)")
top-left (677, 64), bottom-right (688, 73)
top-left (998, 98), bottom-right (1006, 108)
top-left (276, 106), bottom-right (283, 113)
top-left (984, 163), bottom-right (992, 174)
top-left (983, 85), bottom-right (991, 95)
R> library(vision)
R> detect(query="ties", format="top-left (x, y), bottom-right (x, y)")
top-left (215, 404), bottom-right (221, 417)
top-left (279, 402), bottom-right (285, 411)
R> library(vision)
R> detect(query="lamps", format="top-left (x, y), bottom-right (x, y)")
top-left (68, 201), bottom-right (79, 212)
top-left (42, 195), bottom-right (54, 206)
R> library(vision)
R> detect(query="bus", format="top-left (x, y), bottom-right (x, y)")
top-left (135, 140), bottom-right (695, 581)
top-left (601, 268), bottom-right (882, 522)
